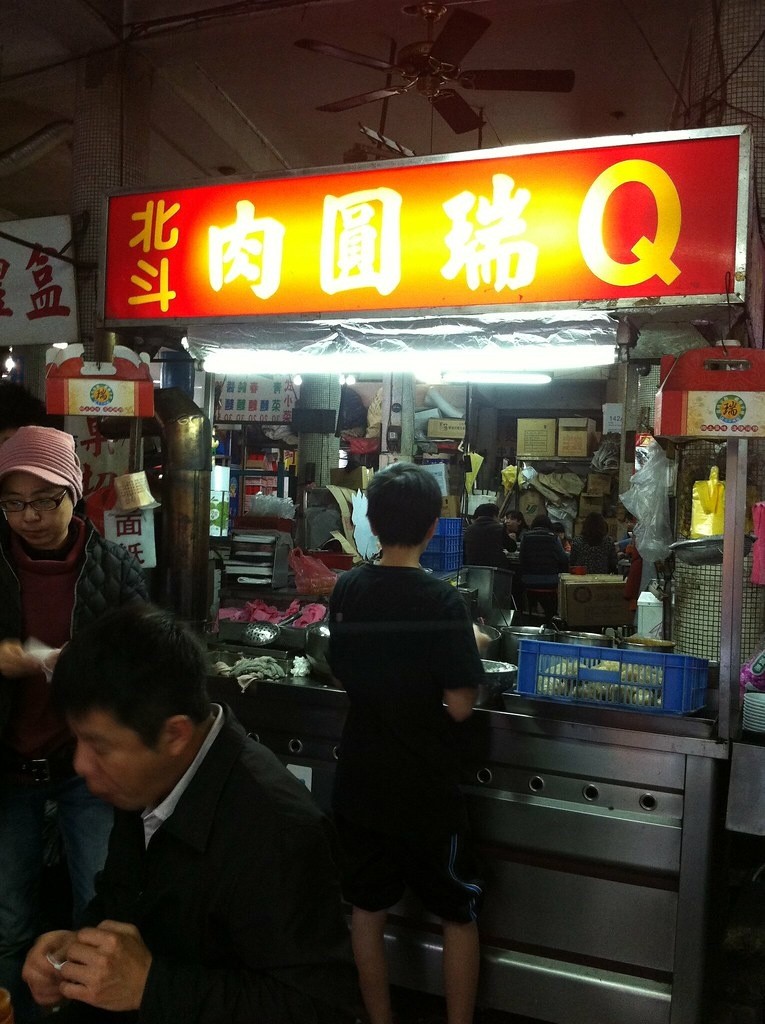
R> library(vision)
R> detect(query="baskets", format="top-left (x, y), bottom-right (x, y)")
top-left (514, 638), bottom-right (709, 717)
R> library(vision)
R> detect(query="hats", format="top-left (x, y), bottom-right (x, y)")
top-left (0, 425), bottom-right (84, 508)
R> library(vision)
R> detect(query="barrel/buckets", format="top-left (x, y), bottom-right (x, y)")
top-left (500, 626), bottom-right (558, 668)
top-left (617, 637), bottom-right (676, 654)
top-left (556, 631), bottom-right (613, 650)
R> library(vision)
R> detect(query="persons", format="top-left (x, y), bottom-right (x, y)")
top-left (321, 461), bottom-right (495, 1024)
top-left (460, 502), bottom-right (644, 630)
top-left (0, 377), bottom-right (151, 935)
top-left (19, 600), bottom-right (356, 1024)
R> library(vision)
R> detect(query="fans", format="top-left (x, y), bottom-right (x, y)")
top-left (292, 1), bottom-right (576, 137)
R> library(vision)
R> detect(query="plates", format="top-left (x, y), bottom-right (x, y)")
top-left (742, 693), bottom-right (765, 733)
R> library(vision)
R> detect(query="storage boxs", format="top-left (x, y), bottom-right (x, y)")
top-left (44, 342), bottom-right (155, 418)
top-left (556, 572), bottom-right (634, 626)
top-left (577, 492), bottom-right (605, 518)
top-left (586, 472), bottom-right (612, 494)
top-left (459, 487), bottom-right (499, 516)
top-left (557, 416), bottom-right (596, 457)
top-left (511, 637), bottom-right (711, 720)
top-left (229, 515), bottom-right (293, 532)
top-left (602, 402), bottom-right (622, 435)
top-left (305, 552), bottom-right (358, 569)
top-left (653, 343), bottom-right (765, 438)
top-left (417, 462), bottom-right (465, 573)
top-left (329, 466), bottom-right (375, 497)
top-left (516, 417), bottom-right (559, 457)
top-left (426, 416), bottom-right (466, 439)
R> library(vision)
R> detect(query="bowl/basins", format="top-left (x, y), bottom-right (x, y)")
top-left (570, 566), bottom-right (587, 575)
top-left (476, 658), bottom-right (518, 707)
top-left (669, 532), bottom-right (756, 566)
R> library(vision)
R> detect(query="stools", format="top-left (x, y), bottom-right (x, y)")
top-left (522, 587), bottom-right (557, 629)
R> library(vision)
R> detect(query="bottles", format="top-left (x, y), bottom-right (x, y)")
top-left (710, 340), bottom-right (748, 372)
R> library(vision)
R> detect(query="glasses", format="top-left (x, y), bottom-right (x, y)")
top-left (0, 488), bottom-right (68, 512)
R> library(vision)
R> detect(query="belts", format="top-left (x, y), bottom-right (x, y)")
top-left (9, 757), bottom-right (69, 783)
